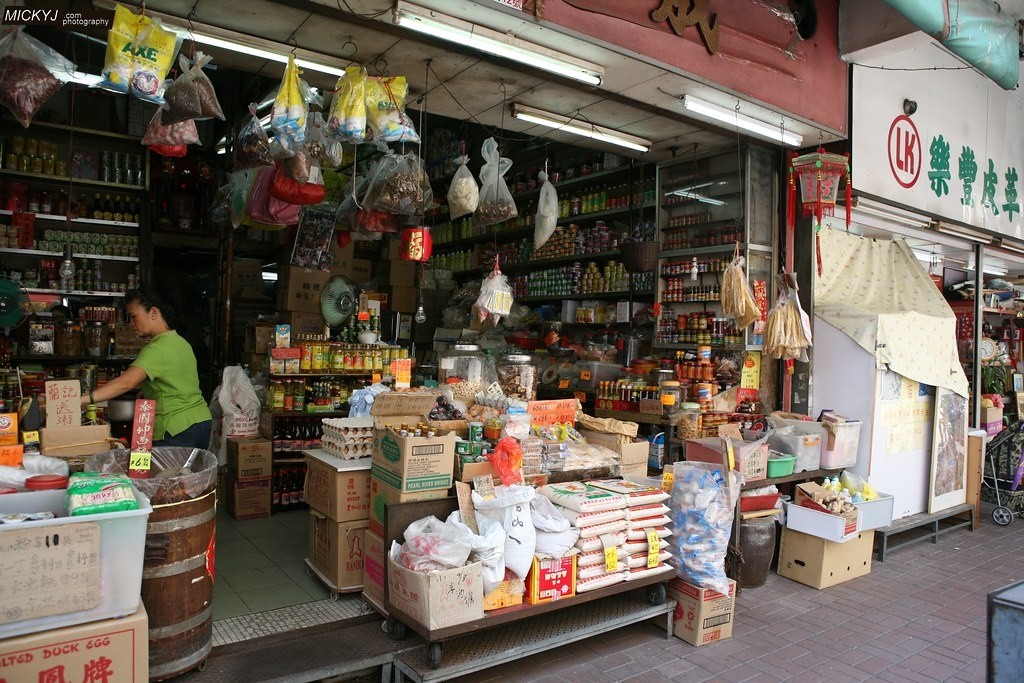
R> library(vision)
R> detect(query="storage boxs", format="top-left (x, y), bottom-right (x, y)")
top-left (386, 549), bottom-right (487, 629)
top-left (302, 457), bottom-right (371, 523)
top-left (0, 443), bottom-right (24, 466)
top-left (0, 411), bottom-right (19, 446)
top-left (0, 596), bottom-right (151, 683)
top-left (856, 485), bottom-right (895, 531)
top-left (786, 500), bottom-right (862, 543)
top-left (742, 426), bottom-right (821, 476)
top-left (224, 476), bottom-right (273, 519)
top-left (226, 436), bottom-right (272, 482)
top-left (796, 481), bottom-right (858, 533)
top-left (369, 476), bottom-right (448, 538)
top-left (969, 404), bottom-right (1005, 436)
top-left (652, 576), bottom-right (743, 646)
top-left (387, 261), bottom-right (418, 286)
top-left (767, 411), bottom-right (867, 470)
top-left (522, 552), bottom-right (577, 607)
top-left (384, 238), bottom-right (402, 259)
top-left (685, 439), bottom-right (768, 484)
top-left (207, 220), bottom-right (387, 369)
top-left (310, 505), bottom-right (371, 588)
top-left (485, 568), bottom-right (529, 613)
top-left (768, 452), bottom-right (797, 479)
top-left (363, 530), bottom-right (386, 610)
top-left (390, 287), bottom-right (415, 313)
top-left (42, 416), bottom-right (113, 457)
top-left (0, 485), bottom-right (159, 640)
top-left (368, 393), bottom-right (459, 494)
top-left (740, 491), bottom-right (783, 510)
top-left (774, 528), bottom-right (877, 591)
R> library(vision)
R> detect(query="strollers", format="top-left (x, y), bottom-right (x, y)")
top-left (982, 418), bottom-right (1024, 527)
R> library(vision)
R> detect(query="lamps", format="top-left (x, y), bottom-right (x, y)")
top-left (91, 0), bottom-right (362, 81)
top-left (960, 261), bottom-right (1009, 278)
top-left (678, 95), bottom-right (804, 148)
top-left (998, 239), bottom-right (1024, 254)
top-left (911, 248), bottom-right (944, 263)
top-left (507, 102), bottom-right (654, 155)
top-left (852, 195), bottom-right (932, 229)
top-left (391, 0), bottom-right (605, 90)
top-left (934, 222), bottom-right (993, 246)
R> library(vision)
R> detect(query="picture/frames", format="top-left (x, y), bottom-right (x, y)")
top-left (927, 385), bottom-right (970, 514)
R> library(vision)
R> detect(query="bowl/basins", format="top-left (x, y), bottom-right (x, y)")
top-left (548, 346), bottom-right (574, 357)
top-left (412, 364), bottom-right (437, 375)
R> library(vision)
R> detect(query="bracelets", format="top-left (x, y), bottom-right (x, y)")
top-left (90, 391), bottom-right (94, 404)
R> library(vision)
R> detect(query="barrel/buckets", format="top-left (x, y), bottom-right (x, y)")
top-left (84, 445), bottom-right (219, 681)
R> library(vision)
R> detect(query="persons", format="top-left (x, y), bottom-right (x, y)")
top-left (81, 287), bottom-right (213, 450)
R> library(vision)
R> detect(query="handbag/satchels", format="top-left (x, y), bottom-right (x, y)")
top-left (225, 111), bottom-right (433, 237)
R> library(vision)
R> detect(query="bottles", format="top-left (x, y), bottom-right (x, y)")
top-left (495, 348), bottom-right (538, 401)
top-left (272, 466), bottom-right (307, 513)
top-left (123, 196), bottom-right (133, 222)
top-left (254, 369), bottom-right (304, 412)
top-left (20, 474), bottom-right (67, 492)
top-left (29, 186), bottom-right (88, 218)
top-left (660, 252), bottom-right (743, 303)
top-left (83, 319), bottom-right (109, 357)
top-left (115, 298), bottom-right (124, 322)
top-left (266, 331), bottom-right (276, 353)
top-left (290, 289), bottom-right (409, 374)
top-left (430, 248), bottom-right (479, 273)
top-left (305, 376), bottom-right (397, 410)
top-left (821, 473), bottom-right (864, 503)
top-left (0, 363), bottom-right (126, 400)
top-left (55, 320), bottom-right (81, 356)
top-left (385, 422), bottom-right (439, 438)
top-left (133, 197), bottom-right (142, 223)
top-left (429, 151), bottom-right (656, 245)
top-left (112, 196), bottom-right (123, 221)
top-left (661, 188), bottom-right (744, 250)
top-left (657, 308), bottom-right (743, 345)
top-left (103, 194), bottom-right (112, 220)
top-left (273, 417), bottom-right (322, 460)
top-left (83, 404), bottom-right (100, 426)
top-left (155, 201), bottom-right (211, 233)
top-left (94, 194), bottom-right (103, 219)
top-left (438, 342), bottom-right (486, 387)
top-left (243, 364), bottom-right (251, 378)
top-left (582, 329), bottom-right (768, 439)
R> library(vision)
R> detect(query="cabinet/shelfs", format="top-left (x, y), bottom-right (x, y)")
top-left (814, 312), bottom-right (969, 520)
top-left (948, 293), bottom-right (1024, 503)
top-left (0, 114), bottom-right (156, 359)
top-left (263, 349), bottom-right (414, 516)
top-left (416, 152), bottom-right (778, 361)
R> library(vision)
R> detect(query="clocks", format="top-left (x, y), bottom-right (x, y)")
top-left (981, 337), bottom-right (997, 361)
